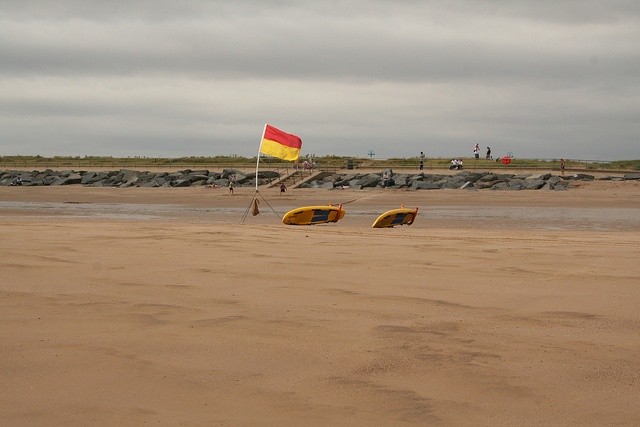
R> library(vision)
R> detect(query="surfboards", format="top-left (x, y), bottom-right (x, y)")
top-left (371, 203), bottom-right (418, 228)
top-left (282, 202), bottom-right (346, 225)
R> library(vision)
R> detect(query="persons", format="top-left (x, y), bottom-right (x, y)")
top-left (448, 158), bottom-right (463, 170)
top-left (560, 158), bottom-right (566, 176)
top-left (14, 177), bottom-right (23, 185)
top-left (229, 180), bottom-right (236, 194)
top-left (473, 143), bottom-right (480, 159)
top-left (419, 151), bottom-right (425, 171)
top-left (279, 182), bottom-right (287, 196)
top-left (486, 147), bottom-right (491, 160)
top-left (382, 168), bottom-right (394, 188)
top-left (332, 177), bottom-right (345, 191)
top-left (294, 159), bottom-right (316, 169)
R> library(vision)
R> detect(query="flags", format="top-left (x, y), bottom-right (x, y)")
top-left (259, 124), bottom-right (302, 161)
